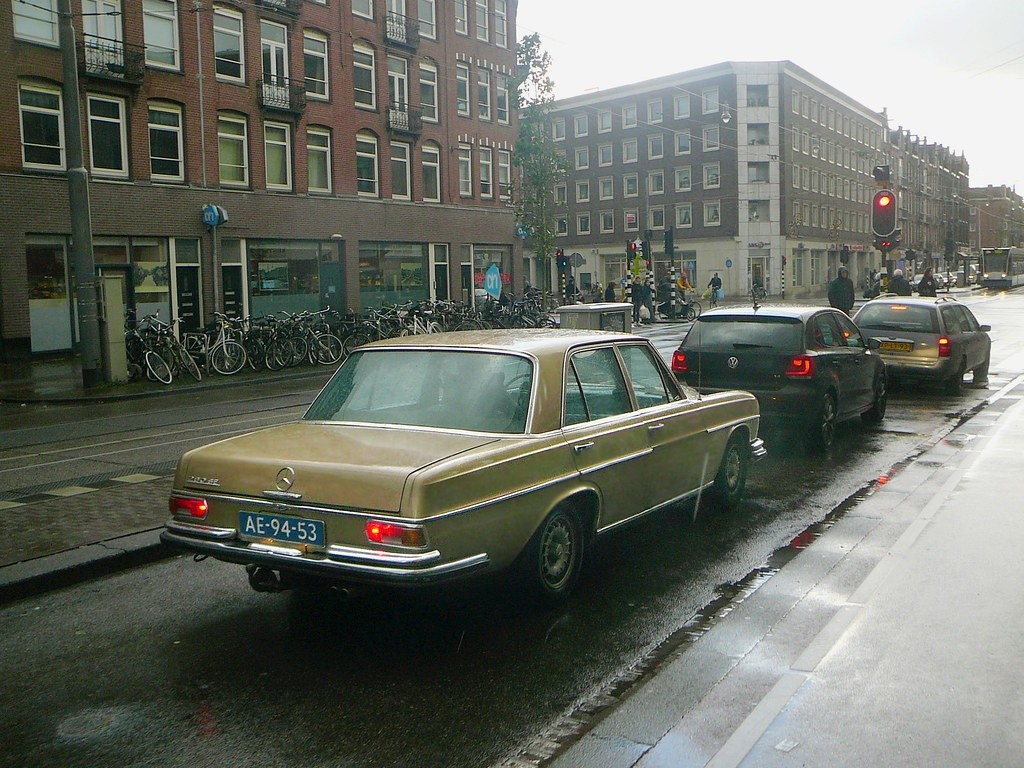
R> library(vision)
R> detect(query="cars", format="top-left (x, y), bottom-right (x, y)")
top-left (909, 264), bottom-right (979, 291)
top-left (853, 291), bottom-right (991, 391)
top-left (162, 328), bottom-right (768, 607)
top-left (671, 280), bottom-right (886, 449)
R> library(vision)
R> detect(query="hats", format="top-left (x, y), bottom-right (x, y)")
top-left (894, 269), bottom-right (902, 276)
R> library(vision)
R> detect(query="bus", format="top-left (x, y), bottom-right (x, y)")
top-left (979, 246), bottom-right (1024, 289)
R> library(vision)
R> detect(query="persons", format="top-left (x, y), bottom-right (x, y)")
top-left (631, 277), bottom-right (643, 326)
top-left (642, 279), bottom-right (657, 321)
top-left (918, 267), bottom-right (937, 297)
top-left (828, 266), bottom-right (855, 315)
top-left (454, 353), bottom-right (496, 375)
top-left (605, 282), bottom-right (616, 302)
top-left (591, 282), bottom-right (603, 301)
top-left (754, 275), bottom-right (763, 291)
top-left (871, 269), bottom-right (880, 283)
top-left (523, 275), bottom-right (529, 287)
top-left (708, 273), bottom-right (722, 303)
top-left (565, 278), bottom-right (579, 298)
top-left (889, 269), bottom-right (911, 295)
top-left (676, 273), bottom-right (694, 302)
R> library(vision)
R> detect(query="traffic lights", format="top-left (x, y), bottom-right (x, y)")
top-left (782, 256), bottom-right (786, 266)
top-left (639, 242), bottom-right (648, 260)
top-left (556, 251), bottom-right (567, 267)
top-left (664, 229), bottom-right (673, 255)
top-left (628, 242), bottom-right (636, 259)
top-left (871, 164), bottom-right (902, 252)
top-left (944, 241), bottom-right (954, 261)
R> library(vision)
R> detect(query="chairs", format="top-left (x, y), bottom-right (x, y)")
top-left (501, 378), bottom-right (531, 435)
top-left (437, 369), bottom-right (507, 432)
top-left (770, 326), bottom-right (796, 349)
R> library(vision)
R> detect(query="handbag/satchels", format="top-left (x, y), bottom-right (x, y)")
top-left (703, 288), bottom-right (713, 298)
top-left (639, 304), bottom-right (651, 320)
top-left (717, 289), bottom-right (725, 301)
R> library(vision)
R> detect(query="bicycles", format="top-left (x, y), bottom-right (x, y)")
top-left (566, 292), bottom-right (583, 305)
top-left (657, 289), bottom-right (702, 321)
top-left (759, 288), bottom-right (768, 302)
top-left (123, 284), bottom-right (559, 385)
top-left (708, 287), bottom-right (719, 309)
